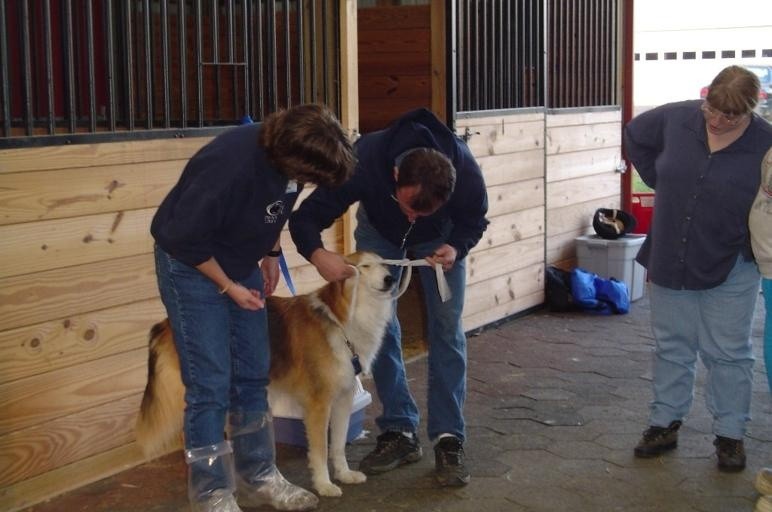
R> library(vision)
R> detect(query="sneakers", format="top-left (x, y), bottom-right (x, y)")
top-left (631, 420), bottom-right (682, 460)
top-left (358, 429), bottom-right (422, 478)
top-left (191, 489), bottom-right (243, 512)
top-left (236, 469), bottom-right (319, 510)
top-left (433, 440), bottom-right (473, 488)
top-left (754, 465), bottom-right (772, 495)
top-left (714, 433), bottom-right (748, 474)
top-left (756, 494), bottom-right (772, 511)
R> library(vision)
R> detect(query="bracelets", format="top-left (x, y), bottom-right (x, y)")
top-left (218, 280), bottom-right (233, 295)
top-left (267, 247), bottom-right (283, 257)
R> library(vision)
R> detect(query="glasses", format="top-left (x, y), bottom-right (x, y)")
top-left (696, 98), bottom-right (749, 127)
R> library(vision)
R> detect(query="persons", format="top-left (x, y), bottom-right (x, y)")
top-left (748, 147), bottom-right (772, 512)
top-left (151, 103), bottom-right (357, 510)
top-left (621, 64), bottom-right (772, 472)
top-left (289, 106), bottom-right (490, 489)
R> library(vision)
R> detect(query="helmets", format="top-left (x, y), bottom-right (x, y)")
top-left (593, 208), bottom-right (636, 239)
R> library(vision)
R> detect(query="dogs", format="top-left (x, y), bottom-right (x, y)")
top-left (135, 249), bottom-right (396, 497)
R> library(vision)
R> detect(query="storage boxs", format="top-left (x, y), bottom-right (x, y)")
top-left (271, 374), bottom-right (373, 449)
top-left (575, 232), bottom-right (649, 303)
